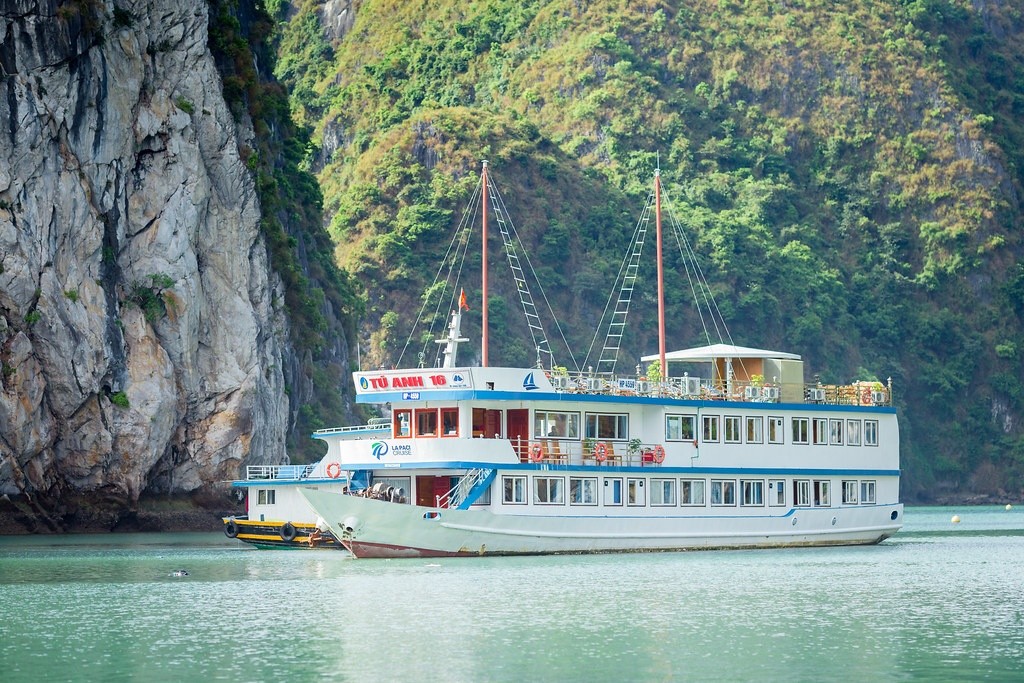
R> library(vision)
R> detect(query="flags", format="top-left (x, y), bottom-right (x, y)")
top-left (458, 287), bottom-right (470, 312)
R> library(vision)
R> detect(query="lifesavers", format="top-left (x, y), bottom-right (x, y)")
top-left (862, 388), bottom-right (871, 404)
top-left (530, 443), bottom-right (544, 462)
top-left (223, 521), bottom-right (239, 538)
top-left (326, 461), bottom-right (341, 479)
top-left (596, 443), bottom-right (608, 462)
top-left (653, 445), bottom-right (665, 463)
top-left (279, 522), bottom-right (297, 542)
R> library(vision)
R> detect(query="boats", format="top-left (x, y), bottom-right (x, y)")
top-left (298, 150), bottom-right (905, 559)
top-left (222, 466), bottom-right (343, 550)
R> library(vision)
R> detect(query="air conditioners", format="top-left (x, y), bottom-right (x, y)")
top-left (765, 387), bottom-right (779, 398)
top-left (871, 392), bottom-right (885, 404)
top-left (745, 386), bottom-right (761, 397)
top-left (681, 377), bottom-right (701, 396)
top-left (586, 378), bottom-right (602, 390)
top-left (555, 377), bottom-right (570, 388)
top-left (637, 381), bottom-right (652, 394)
top-left (811, 389), bottom-right (827, 400)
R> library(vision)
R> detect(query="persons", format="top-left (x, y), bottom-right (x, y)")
top-left (548, 427), bottom-right (558, 436)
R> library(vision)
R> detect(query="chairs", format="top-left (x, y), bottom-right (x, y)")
top-left (583, 440), bottom-right (623, 467)
top-left (539, 439), bottom-right (568, 465)
